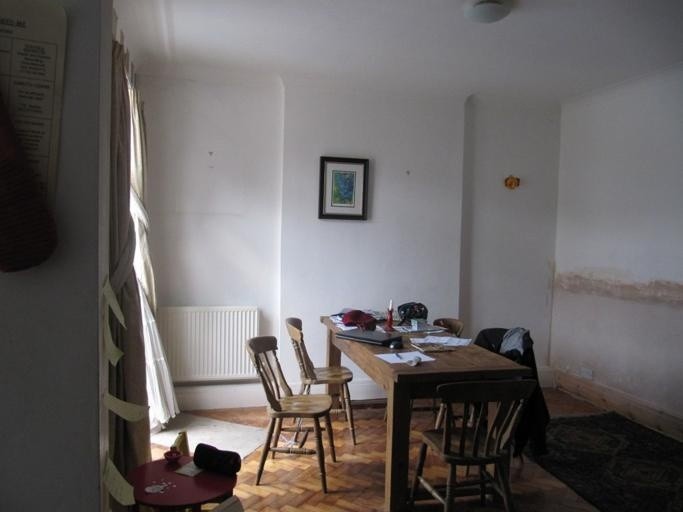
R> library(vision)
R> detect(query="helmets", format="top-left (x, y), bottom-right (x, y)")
top-left (398, 301), bottom-right (428, 326)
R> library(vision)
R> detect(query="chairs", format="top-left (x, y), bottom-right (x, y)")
top-left (246, 317), bottom-right (356, 495)
top-left (410, 315), bottom-right (534, 512)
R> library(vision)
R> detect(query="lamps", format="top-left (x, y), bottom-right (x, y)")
top-left (464, 1), bottom-right (510, 25)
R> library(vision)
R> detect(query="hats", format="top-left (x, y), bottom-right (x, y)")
top-left (342, 310), bottom-right (375, 326)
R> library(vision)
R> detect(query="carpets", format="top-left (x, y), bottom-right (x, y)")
top-left (521, 412), bottom-right (683, 511)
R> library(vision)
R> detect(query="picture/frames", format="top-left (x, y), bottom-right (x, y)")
top-left (318, 155), bottom-right (369, 220)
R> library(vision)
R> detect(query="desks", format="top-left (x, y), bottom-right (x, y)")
top-left (320, 315), bottom-right (532, 511)
top-left (125, 456), bottom-right (238, 512)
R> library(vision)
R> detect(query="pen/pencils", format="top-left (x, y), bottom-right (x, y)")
top-left (394, 351), bottom-right (402, 359)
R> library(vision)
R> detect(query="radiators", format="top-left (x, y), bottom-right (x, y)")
top-left (155, 306), bottom-right (259, 385)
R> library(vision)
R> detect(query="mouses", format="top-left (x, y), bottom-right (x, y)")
top-left (390, 342), bottom-right (403, 349)
